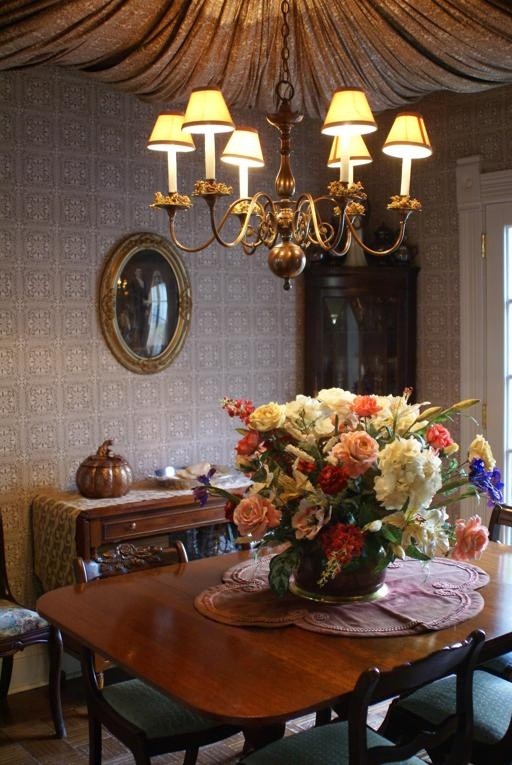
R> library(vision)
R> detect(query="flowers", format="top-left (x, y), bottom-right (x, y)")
top-left (191, 385), bottom-right (512, 601)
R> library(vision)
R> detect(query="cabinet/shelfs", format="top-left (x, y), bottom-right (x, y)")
top-left (303, 266), bottom-right (421, 413)
top-left (34, 470), bottom-right (275, 692)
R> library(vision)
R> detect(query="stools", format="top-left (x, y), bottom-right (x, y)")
top-left (476, 644), bottom-right (512, 681)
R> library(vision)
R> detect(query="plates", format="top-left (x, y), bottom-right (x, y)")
top-left (148, 464), bottom-right (243, 488)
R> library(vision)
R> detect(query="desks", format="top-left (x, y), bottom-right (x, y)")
top-left (35, 541), bottom-right (512, 761)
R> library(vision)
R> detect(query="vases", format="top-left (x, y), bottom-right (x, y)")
top-left (294, 546), bottom-right (387, 596)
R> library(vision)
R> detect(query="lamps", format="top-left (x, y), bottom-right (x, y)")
top-left (144, 1), bottom-right (435, 290)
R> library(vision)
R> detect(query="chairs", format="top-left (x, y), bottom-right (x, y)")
top-left (377, 663), bottom-right (512, 764)
top-left (71, 538), bottom-right (267, 765)
top-left (479, 502), bottom-right (512, 545)
top-left (0, 513), bottom-right (67, 737)
top-left (237, 627), bottom-right (488, 765)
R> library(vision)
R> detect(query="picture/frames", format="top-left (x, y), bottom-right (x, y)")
top-left (100, 231), bottom-right (194, 375)
